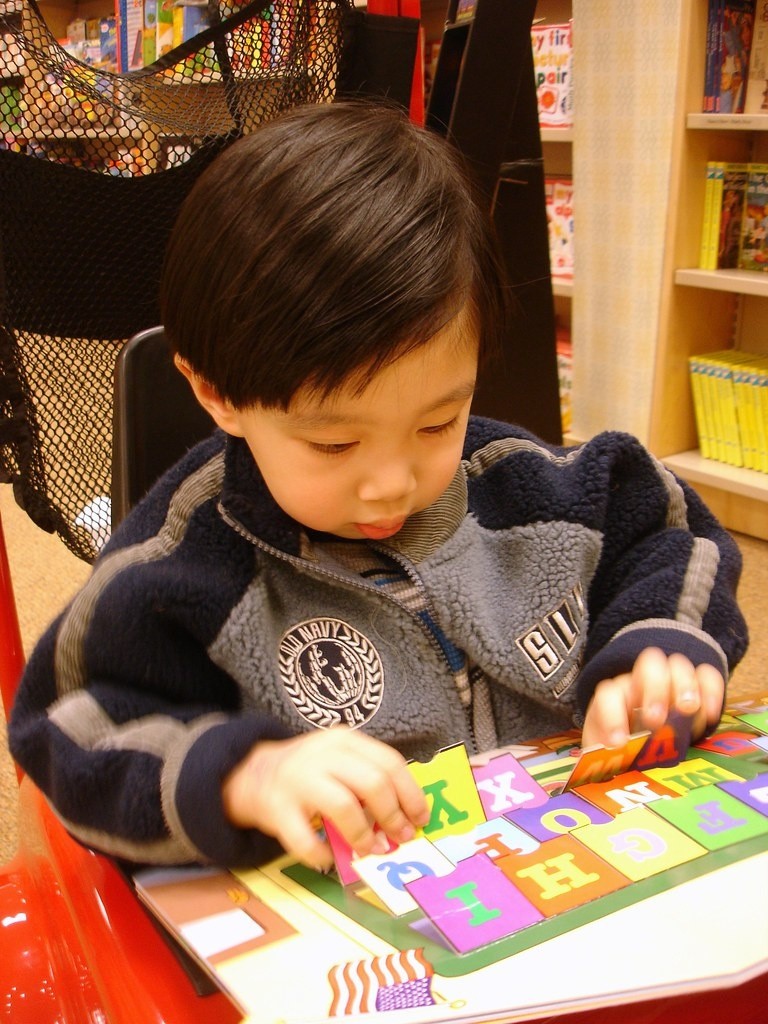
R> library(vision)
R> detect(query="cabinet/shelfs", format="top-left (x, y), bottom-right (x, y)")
top-left (0, 0), bottom-right (768, 544)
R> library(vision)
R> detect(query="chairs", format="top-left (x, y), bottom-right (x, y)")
top-left (112, 325), bottom-right (217, 530)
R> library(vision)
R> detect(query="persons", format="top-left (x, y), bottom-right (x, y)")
top-left (8, 100), bottom-right (749, 872)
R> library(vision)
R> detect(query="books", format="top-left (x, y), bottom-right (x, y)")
top-left (705, 0), bottom-right (768, 112)
top-left (541, 176), bottom-right (573, 280)
top-left (529, 24), bottom-right (571, 125)
top-left (689, 349), bottom-right (768, 473)
top-left (0, 139), bottom-right (140, 179)
top-left (121, 1), bottom-right (332, 83)
top-left (701, 162), bottom-right (767, 274)
top-left (0, 16), bottom-right (120, 128)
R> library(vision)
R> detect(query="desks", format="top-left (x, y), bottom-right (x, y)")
top-left (133, 689), bottom-right (768, 1024)
